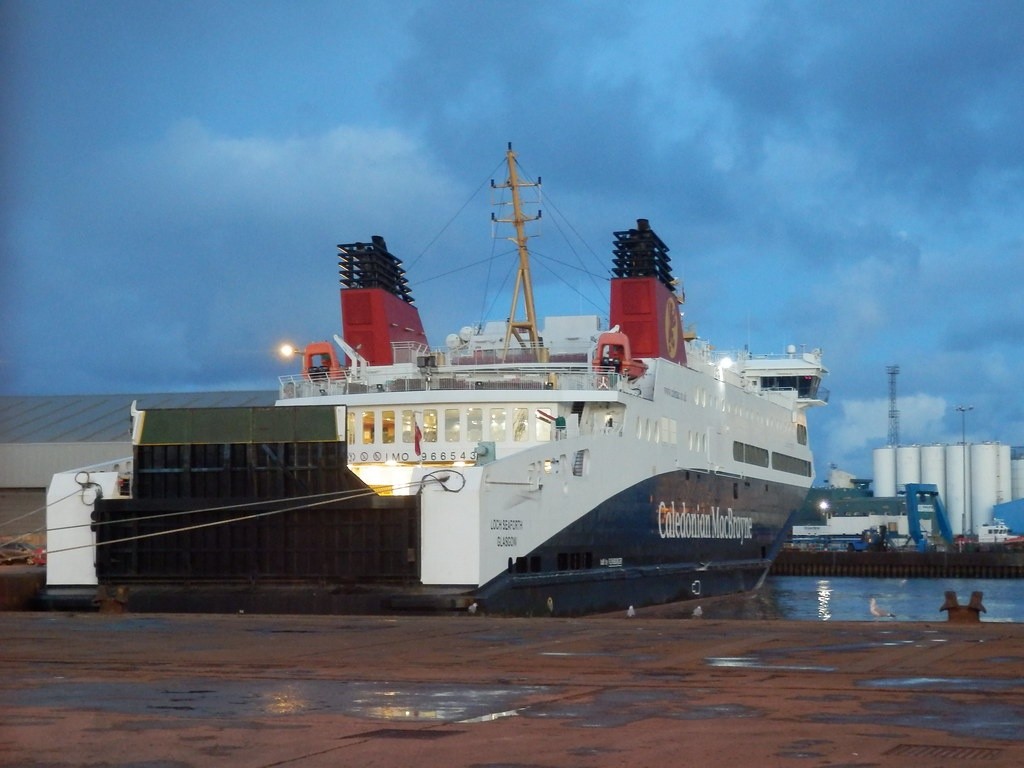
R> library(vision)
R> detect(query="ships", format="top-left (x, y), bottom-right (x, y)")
top-left (43, 135), bottom-right (831, 614)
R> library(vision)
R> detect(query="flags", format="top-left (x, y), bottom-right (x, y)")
top-left (415, 421), bottom-right (422, 456)
